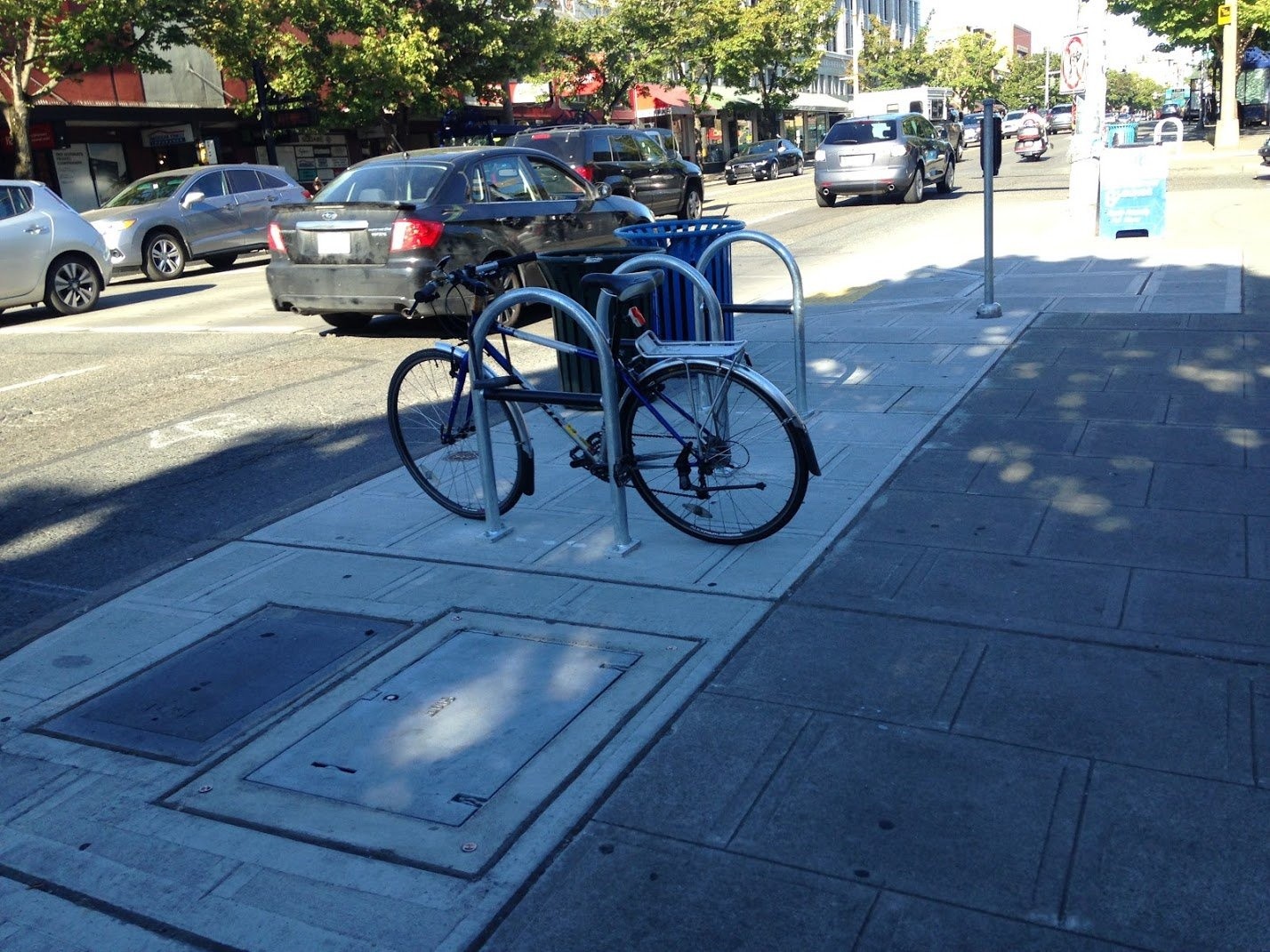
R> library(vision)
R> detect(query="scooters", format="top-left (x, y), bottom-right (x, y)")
top-left (1015, 120), bottom-right (1052, 162)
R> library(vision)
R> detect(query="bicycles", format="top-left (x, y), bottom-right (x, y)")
top-left (388, 249), bottom-right (823, 546)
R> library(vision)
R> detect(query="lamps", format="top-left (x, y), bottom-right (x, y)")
top-left (184, 61), bottom-right (241, 107)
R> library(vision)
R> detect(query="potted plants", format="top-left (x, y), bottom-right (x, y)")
top-left (1107, 118), bottom-right (1139, 148)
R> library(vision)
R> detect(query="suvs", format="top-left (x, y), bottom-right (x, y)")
top-left (1048, 104), bottom-right (1074, 134)
top-left (636, 128), bottom-right (682, 162)
top-left (1160, 104), bottom-right (1181, 118)
top-left (814, 112), bottom-right (956, 208)
top-left (487, 122), bottom-right (705, 219)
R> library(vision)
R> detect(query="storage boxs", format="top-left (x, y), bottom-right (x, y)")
top-left (1016, 127), bottom-right (1042, 140)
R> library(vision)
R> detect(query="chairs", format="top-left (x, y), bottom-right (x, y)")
top-left (360, 187), bottom-right (389, 203)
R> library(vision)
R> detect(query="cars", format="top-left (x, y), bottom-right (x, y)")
top-left (1106, 111), bottom-right (1153, 124)
top-left (724, 138), bottom-right (804, 187)
top-left (79, 164), bottom-right (315, 281)
top-left (961, 111), bottom-right (1003, 147)
top-left (265, 146), bottom-right (657, 336)
top-left (1002, 108), bottom-right (1052, 139)
top-left (0, 179), bottom-right (112, 318)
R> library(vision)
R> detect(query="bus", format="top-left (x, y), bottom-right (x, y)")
top-left (850, 87), bottom-right (954, 136)
top-left (1165, 87), bottom-right (1190, 110)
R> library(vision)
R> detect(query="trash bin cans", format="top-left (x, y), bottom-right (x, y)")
top-left (612, 220), bottom-right (747, 377)
top-left (1105, 121), bottom-right (1138, 148)
top-left (535, 246), bottom-right (666, 410)
